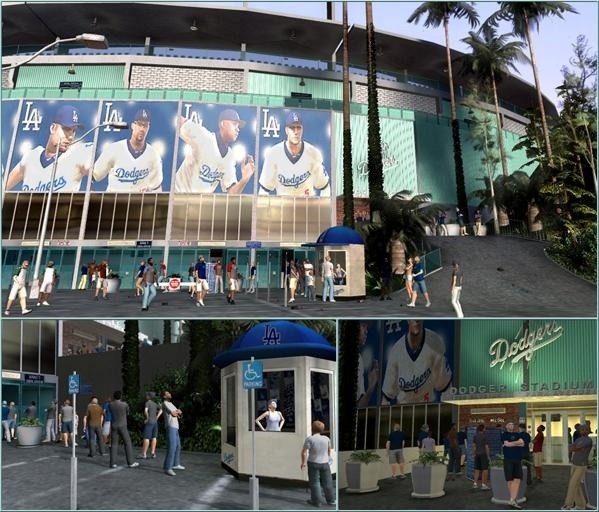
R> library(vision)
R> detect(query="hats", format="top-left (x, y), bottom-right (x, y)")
top-left (134, 107), bottom-right (151, 121)
top-left (287, 112), bottom-right (301, 125)
top-left (54, 106), bottom-right (83, 131)
top-left (219, 109), bottom-right (246, 129)
